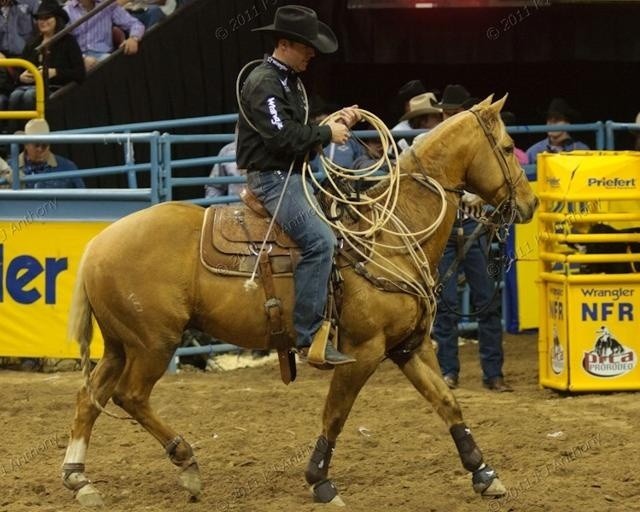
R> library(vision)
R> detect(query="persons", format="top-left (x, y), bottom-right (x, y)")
top-left (198, 79), bottom-right (596, 199)
top-left (230, 4), bottom-right (364, 367)
top-left (427, 85), bottom-right (518, 394)
top-left (0, 1), bottom-right (188, 192)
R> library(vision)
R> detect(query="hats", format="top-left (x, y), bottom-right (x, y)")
top-left (14, 118), bottom-right (50, 134)
top-left (31, 0), bottom-right (69, 24)
top-left (397, 80), bottom-right (475, 122)
top-left (537, 98), bottom-right (579, 117)
top-left (250, 4), bottom-right (339, 54)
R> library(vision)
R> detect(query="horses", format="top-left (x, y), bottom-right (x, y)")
top-left (58, 90), bottom-right (542, 510)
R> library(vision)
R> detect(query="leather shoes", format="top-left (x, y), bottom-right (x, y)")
top-left (483, 375), bottom-right (514, 392)
top-left (299, 339), bottom-right (357, 364)
top-left (442, 373), bottom-right (458, 388)
